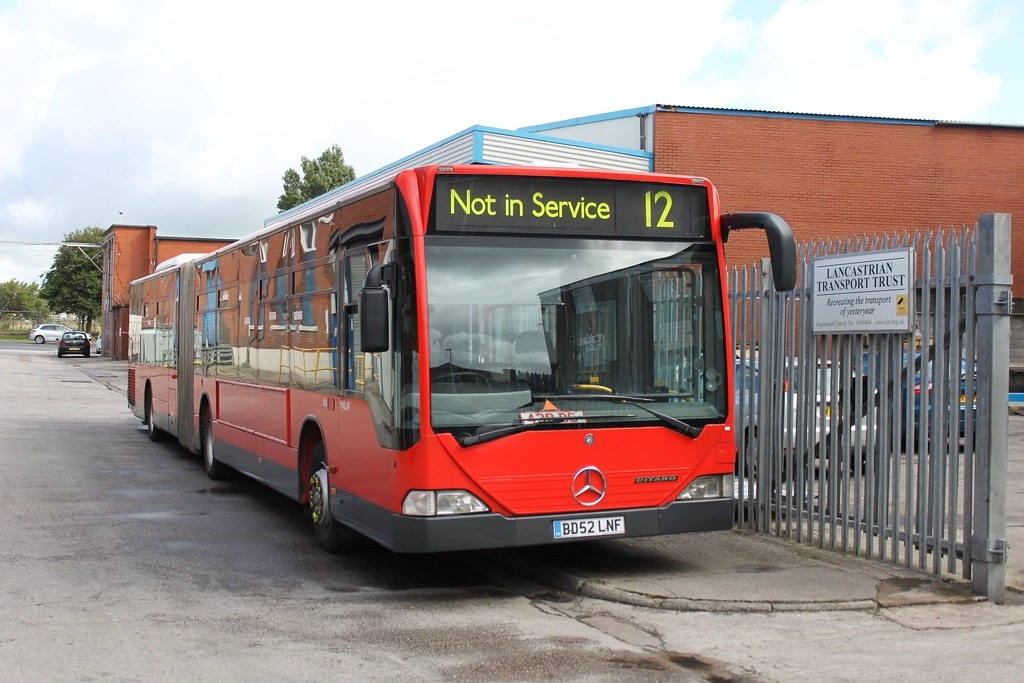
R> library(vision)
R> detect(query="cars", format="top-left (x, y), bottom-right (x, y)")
top-left (54, 330), bottom-right (92, 358)
top-left (734, 354), bottom-right (880, 479)
top-left (94, 331), bottom-right (102, 354)
top-left (839, 348), bottom-right (980, 452)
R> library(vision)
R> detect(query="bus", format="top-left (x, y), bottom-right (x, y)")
top-left (122, 160), bottom-right (799, 556)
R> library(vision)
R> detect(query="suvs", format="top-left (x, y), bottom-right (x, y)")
top-left (29, 322), bottom-right (93, 344)
